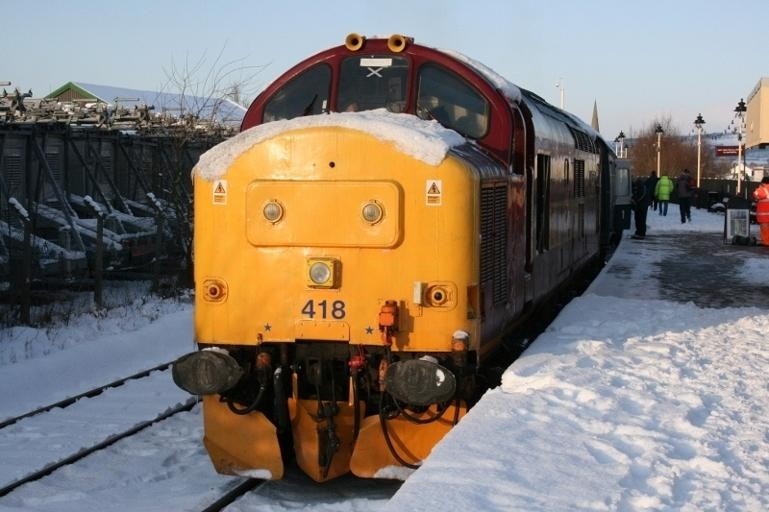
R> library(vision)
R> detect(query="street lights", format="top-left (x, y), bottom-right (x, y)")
top-left (554, 82), bottom-right (565, 110)
top-left (655, 124), bottom-right (664, 178)
top-left (733, 98), bottom-right (747, 196)
top-left (693, 113), bottom-right (706, 187)
top-left (613, 130), bottom-right (628, 158)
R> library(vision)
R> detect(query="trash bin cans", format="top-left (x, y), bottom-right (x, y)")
top-left (724, 199), bottom-right (751, 243)
top-left (708, 192), bottom-right (719, 212)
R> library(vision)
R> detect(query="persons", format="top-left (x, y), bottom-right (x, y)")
top-left (672, 168), bottom-right (692, 223)
top-left (647, 171), bottom-right (660, 210)
top-left (630, 180), bottom-right (649, 240)
top-left (751, 176), bottom-right (769, 247)
top-left (655, 175), bottom-right (673, 216)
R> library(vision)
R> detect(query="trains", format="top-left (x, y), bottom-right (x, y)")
top-left (172, 32), bottom-right (631, 481)
